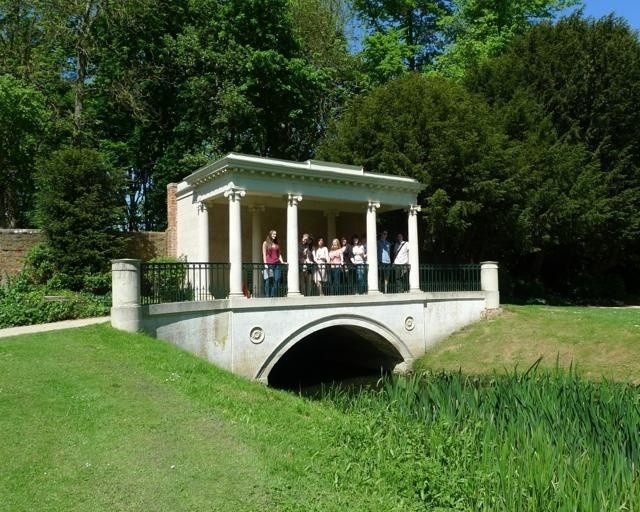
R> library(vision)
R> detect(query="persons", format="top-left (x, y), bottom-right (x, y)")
top-left (391, 233), bottom-right (408, 293)
top-left (376, 229), bottom-right (393, 293)
top-left (298, 232), bottom-right (368, 296)
top-left (262, 230), bottom-right (287, 297)
